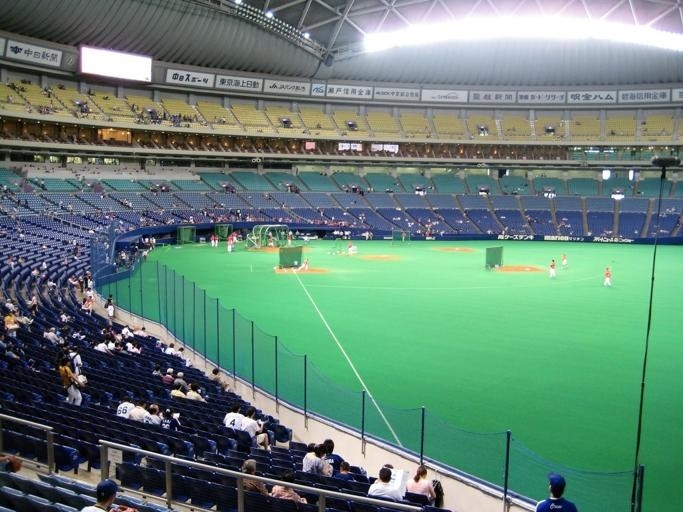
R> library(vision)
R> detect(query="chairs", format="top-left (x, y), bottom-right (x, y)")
top-left (0, 225), bottom-right (444, 512)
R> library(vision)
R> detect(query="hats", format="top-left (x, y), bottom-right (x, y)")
top-left (546, 472), bottom-right (565, 490)
top-left (96, 479), bottom-right (123, 496)
